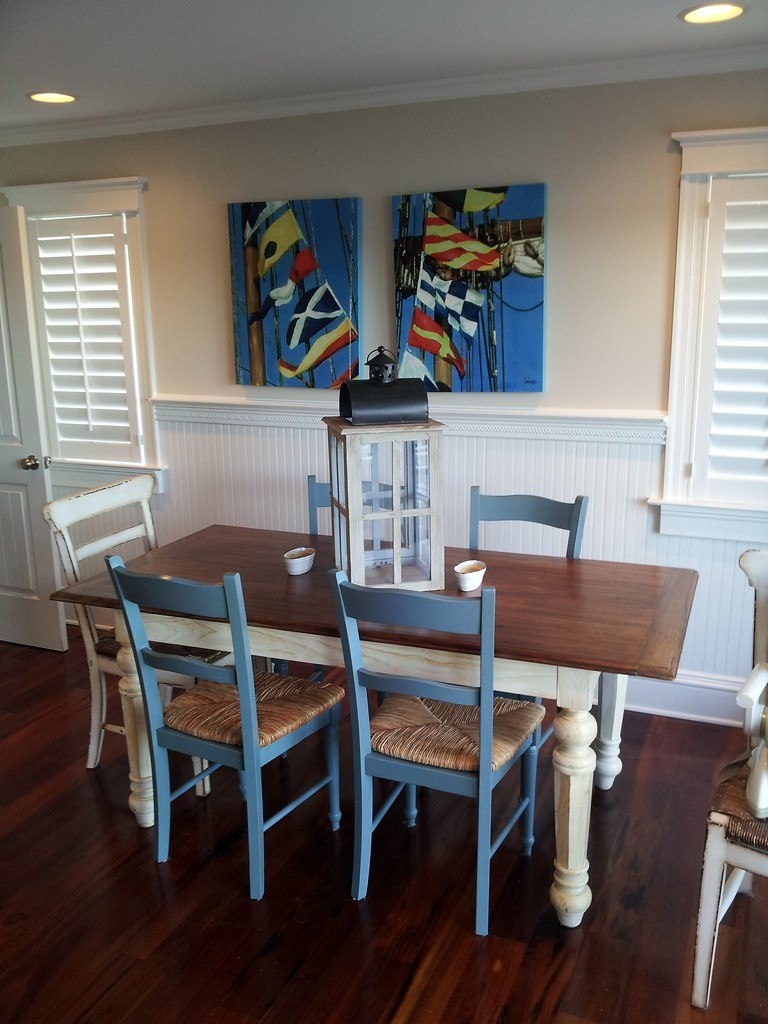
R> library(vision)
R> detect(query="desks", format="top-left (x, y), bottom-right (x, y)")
top-left (48, 523), bottom-right (701, 930)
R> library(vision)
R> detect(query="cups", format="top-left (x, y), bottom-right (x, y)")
top-left (453, 559), bottom-right (487, 592)
top-left (282, 547), bottom-right (316, 575)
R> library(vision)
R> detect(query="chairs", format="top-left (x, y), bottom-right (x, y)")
top-left (101, 553), bottom-right (346, 902)
top-left (275, 475), bottom-right (415, 686)
top-left (471, 487), bottom-right (589, 760)
top-left (691, 547), bottom-right (768, 1012)
top-left (40, 472), bottom-right (239, 795)
top-left (326, 569), bottom-right (545, 936)
